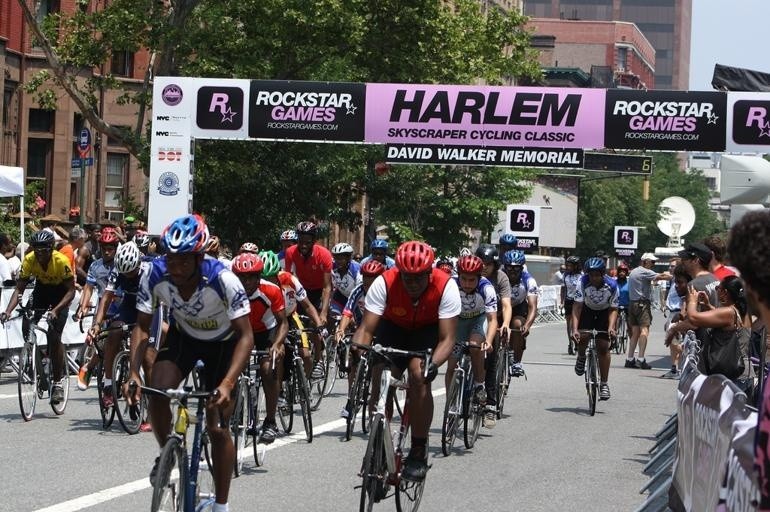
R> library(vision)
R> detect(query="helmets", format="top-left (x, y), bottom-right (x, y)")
top-left (231, 221), bottom-right (388, 276)
top-left (564, 254), bottom-right (584, 267)
top-left (159, 214), bottom-right (209, 254)
top-left (617, 264), bottom-right (630, 274)
top-left (395, 241), bottom-right (434, 276)
top-left (29, 229), bottom-right (56, 249)
top-left (581, 254), bottom-right (609, 274)
top-left (204, 236), bottom-right (220, 253)
top-left (435, 233), bottom-right (525, 272)
top-left (98, 225), bottom-right (150, 274)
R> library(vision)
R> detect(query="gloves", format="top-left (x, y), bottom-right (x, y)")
top-left (338, 347), bottom-right (358, 372)
top-left (422, 360), bottom-right (439, 385)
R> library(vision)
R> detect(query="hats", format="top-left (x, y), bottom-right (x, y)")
top-left (676, 239), bottom-right (714, 268)
top-left (638, 251), bottom-right (660, 263)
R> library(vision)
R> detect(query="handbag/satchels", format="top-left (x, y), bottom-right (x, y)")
top-left (696, 321), bottom-right (747, 381)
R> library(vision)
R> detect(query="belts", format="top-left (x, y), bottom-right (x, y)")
top-left (668, 308), bottom-right (681, 313)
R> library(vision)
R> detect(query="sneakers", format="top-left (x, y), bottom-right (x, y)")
top-left (309, 359), bottom-right (326, 380)
top-left (149, 451), bottom-right (178, 490)
top-left (661, 370), bottom-right (682, 381)
top-left (140, 412), bottom-right (153, 433)
top-left (573, 354), bottom-right (587, 377)
top-left (76, 361), bottom-right (93, 391)
top-left (624, 356), bottom-right (652, 369)
top-left (599, 382), bottom-right (611, 402)
top-left (259, 416), bottom-right (280, 444)
top-left (342, 398), bottom-right (355, 420)
top-left (511, 362), bottom-right (526, 376)
top-left (100, 383), bottom-right (118, 409)
top-left (401, 441), bottom-right (431, 482)
top-left (483, 405), bottom-right (498, 428)
top-left (474, 383), bottom-right (489, 404)
top-left (49, 384), bottom-right (66, 403)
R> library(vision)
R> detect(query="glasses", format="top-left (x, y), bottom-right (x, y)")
top-left (504, 263), bottom-right (523, 272)
top-left (714, 285), bottom-right (726, 292)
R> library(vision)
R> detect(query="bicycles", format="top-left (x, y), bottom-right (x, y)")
top-left (570, 328), bottom-right (617, 416)
top-left (123, 379), bottom-right (216, 511)
top-left (339, 335), bottom-right (434, 512)
top-left (615, 304), bottom-right (628, 355)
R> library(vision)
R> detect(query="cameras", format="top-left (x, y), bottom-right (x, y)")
top-left (698, 294), bottom-right (704, 301)
